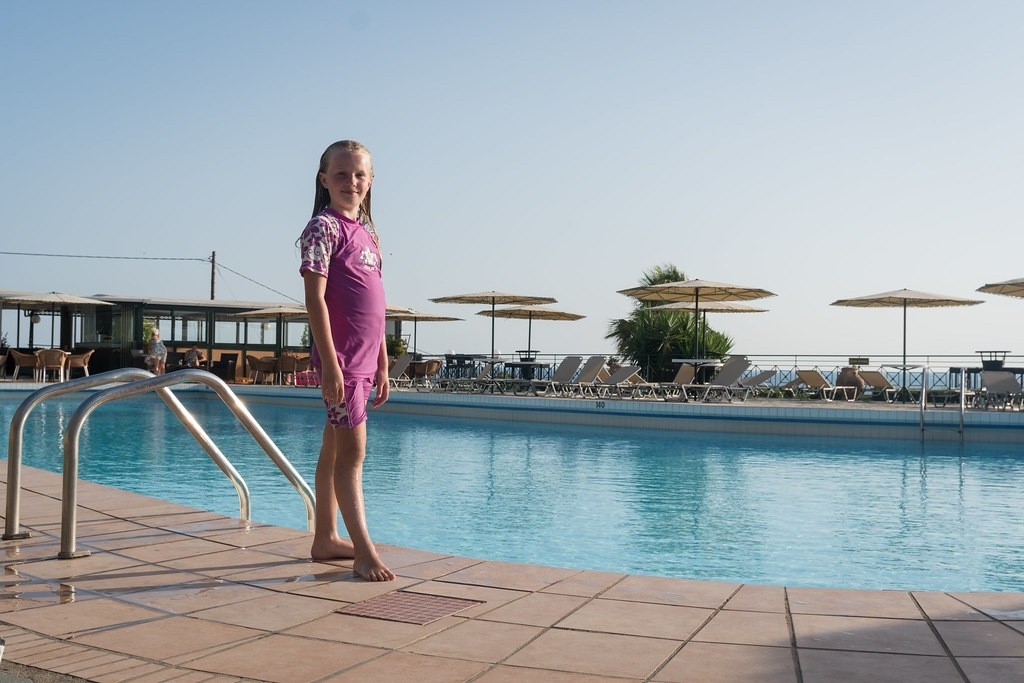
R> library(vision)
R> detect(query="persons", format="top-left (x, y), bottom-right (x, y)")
top-left (184, 344), bottom-right (206, 367)
top-left (145, 328), bottom-right (169, 376)
top-left (294, 139), bottom-right (399, 585)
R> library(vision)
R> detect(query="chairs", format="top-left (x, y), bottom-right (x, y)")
top-left (2, 329), bottom-right (1022, 411)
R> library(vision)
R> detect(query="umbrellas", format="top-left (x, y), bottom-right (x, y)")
top-left (384, 309), bottom-right (466, 388)
top-left (615, 278), bottom-right (779, 402)
top-left (474, 304), bottom-right (588, 390)
top-left (383, 305), bottom-right (414, 317)
top-left (226, 305), bottom-right (309, 384)
top-left (830, 287), bottom-right (986, 404)
top-left (975, 277), bottom-right (1024, 300)
top-left (429, 289), bottom-right (558, 394)
top-left (2, 290), bottom-right (118, 382)
top-left (644, 301), bottom-right (770, 399)
